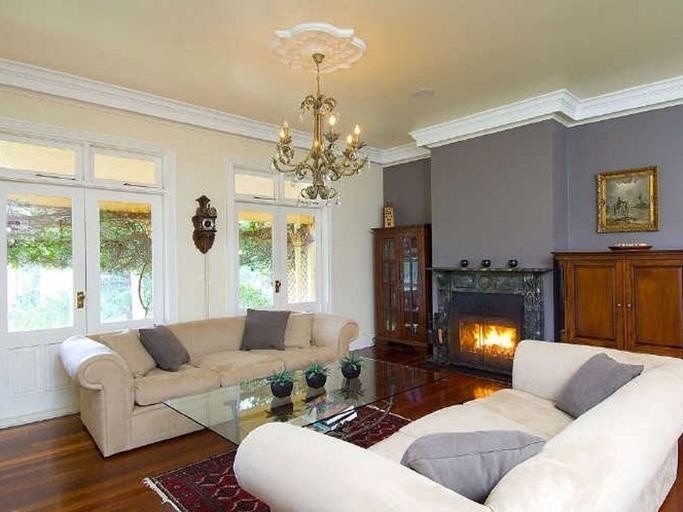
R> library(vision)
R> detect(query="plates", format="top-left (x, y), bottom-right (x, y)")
top-left (609, 243), bottom-right (654, 250)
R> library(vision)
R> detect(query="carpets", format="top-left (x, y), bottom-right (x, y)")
top-left (136, 402), bottom-right (415, 510)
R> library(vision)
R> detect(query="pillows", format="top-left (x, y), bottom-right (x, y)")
top-left (400, 427), bottom-right (546, 502)
top-left (283, 310), bottom-right (313, 350)
top-left (100, 328), bottom-right (157, 377)
top-left (551, 352), bottom-right (643, 419)
top-left (239, 308), bottom-right (290, 352)
top-left (138, 323), bottom-right (190, 371)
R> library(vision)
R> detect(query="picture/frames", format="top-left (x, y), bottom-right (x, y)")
top-left (595, 163), bottom-right (660, 234)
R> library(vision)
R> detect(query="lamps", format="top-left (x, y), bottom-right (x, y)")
top-left (268, 19), bottom-right (372, 204)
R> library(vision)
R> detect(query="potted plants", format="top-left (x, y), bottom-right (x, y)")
top-left (302, 363), bottom-right (326, 389)
top-left (337, 354), bottom-right (365, 379)
top-left (266, 367), bottom-right (295, 398)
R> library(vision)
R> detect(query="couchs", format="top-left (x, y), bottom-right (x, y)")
top-left (234, 337), bottom-right (681, 512)
top-left (58, 310), bottom-right (359, 459)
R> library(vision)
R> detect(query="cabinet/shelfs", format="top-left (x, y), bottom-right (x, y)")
top-left (368, 223), bottom-right (433, 359)
top-left (548, 247), bottom-right (682, 358)
